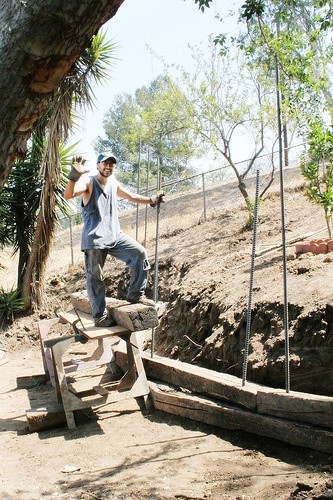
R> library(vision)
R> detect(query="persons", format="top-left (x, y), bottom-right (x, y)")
top-left (64, 152), bottom-right (166, 327)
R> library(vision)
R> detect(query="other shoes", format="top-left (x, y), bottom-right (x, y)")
top-left (126, 291), bottom-right (155, 306)
top-left (94, 312), bottom-right (115, 326)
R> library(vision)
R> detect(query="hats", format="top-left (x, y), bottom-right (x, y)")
top-left (97, 152), bottom-right (116, 164)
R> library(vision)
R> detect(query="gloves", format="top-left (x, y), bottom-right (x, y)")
top-left (150, 191), bottom-right (165, 207)
top-left (67, 154), bottom-right (90, 182)
